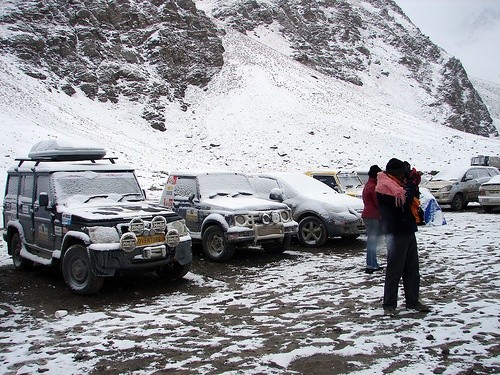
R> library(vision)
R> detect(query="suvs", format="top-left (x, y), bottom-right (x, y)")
top-left (2, 140), bottom-right (194, 296)
top-left (158, 172), bottom-right (299, 264)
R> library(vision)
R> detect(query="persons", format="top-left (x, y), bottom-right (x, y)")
top-left (361, 165), bottom-right (382, 274)
top-left (374, 158), bottom-right (428, 316)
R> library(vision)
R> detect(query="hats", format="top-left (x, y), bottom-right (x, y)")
top-left (367, 165), bottom-right (383, 176)
top-left (386, 157), bottom-right (405, 176)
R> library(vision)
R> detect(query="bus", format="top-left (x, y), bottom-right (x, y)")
top-left (470, 154), bottom-right (500, 170)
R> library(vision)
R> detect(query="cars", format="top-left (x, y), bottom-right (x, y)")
top-left (304, 169), bottom-right (438, 231)
top-left (240, 175), bottom-right (370, 246)
top-left (424, 165), bottom-right (500, 210)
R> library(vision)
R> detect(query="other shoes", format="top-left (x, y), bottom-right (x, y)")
top-left (384, 305), bottom-right (395, 316)
top-left (366, 268), bottom-right (373, 274)
top-left (373, 266), bottom-right (384, 271)
top-left (406, 301), bottom-right (429, 312)
